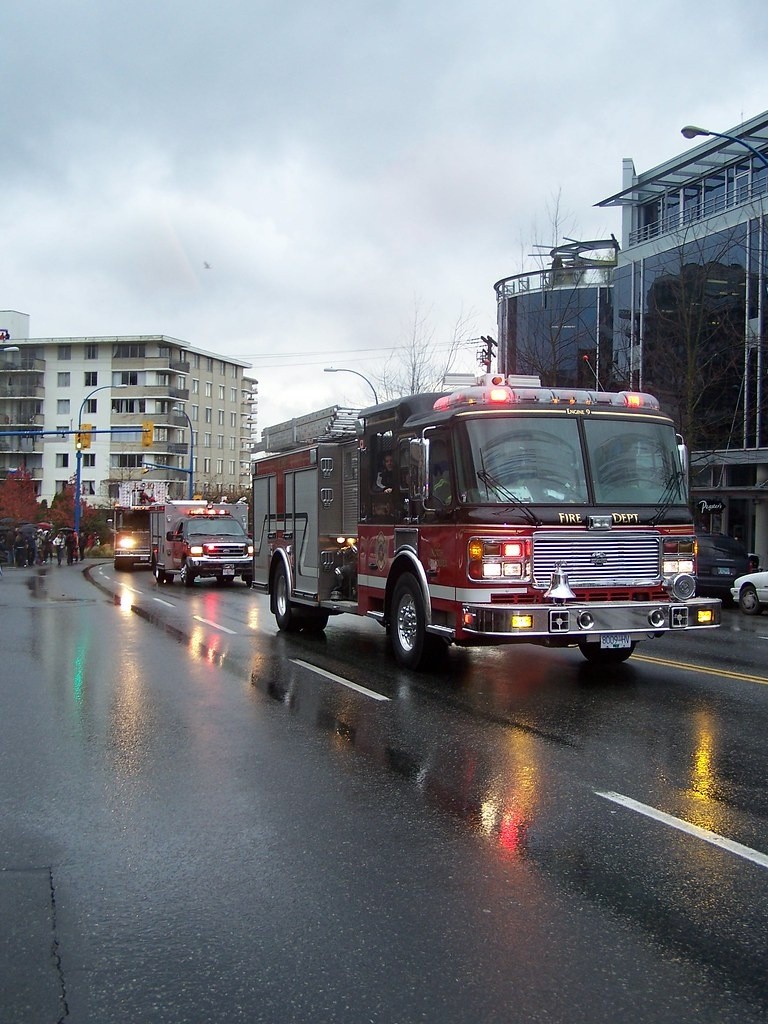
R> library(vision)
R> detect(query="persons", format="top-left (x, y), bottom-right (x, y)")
top-left (0, 526), bottom-right (101, 575)
top-left (377, 452), bottom-right (410, 493)
top-left (433, 460), bottom-right (451, 504)
top-left (695, 518), bottom-right (708, 533)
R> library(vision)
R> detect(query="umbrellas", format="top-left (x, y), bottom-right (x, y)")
top-left (58, 527), bottom-right (75, 536)
top-left (0, 518), bottom-right (54, 537)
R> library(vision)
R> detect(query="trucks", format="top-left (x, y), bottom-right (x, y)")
top-left (106, 502), bottom-right (153, 571)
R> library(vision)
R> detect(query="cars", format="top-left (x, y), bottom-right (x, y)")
top-left (693, 532), bottom-right (758, 615)
top-left (730, 571), bottom-right (768, 616)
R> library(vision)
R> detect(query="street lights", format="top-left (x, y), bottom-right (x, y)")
top-left (172, 406), bottom-right (194, 499)
top-left (74, 384), bottom-right (129, 537)
top-left (324, 368), bottom-right (378, 406)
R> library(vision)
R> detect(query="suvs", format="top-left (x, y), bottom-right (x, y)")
top-left (155, 508), bottom-right (255, 586)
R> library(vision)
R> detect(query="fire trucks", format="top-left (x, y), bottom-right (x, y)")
top-left (250, 372), bottom-right (723, 669)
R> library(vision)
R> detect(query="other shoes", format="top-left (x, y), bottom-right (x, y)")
top-left (41, 561), bottom-right (45, 566)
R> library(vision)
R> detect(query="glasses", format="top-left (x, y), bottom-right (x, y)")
top-left (433, 470), bottom-right (443, 476)
